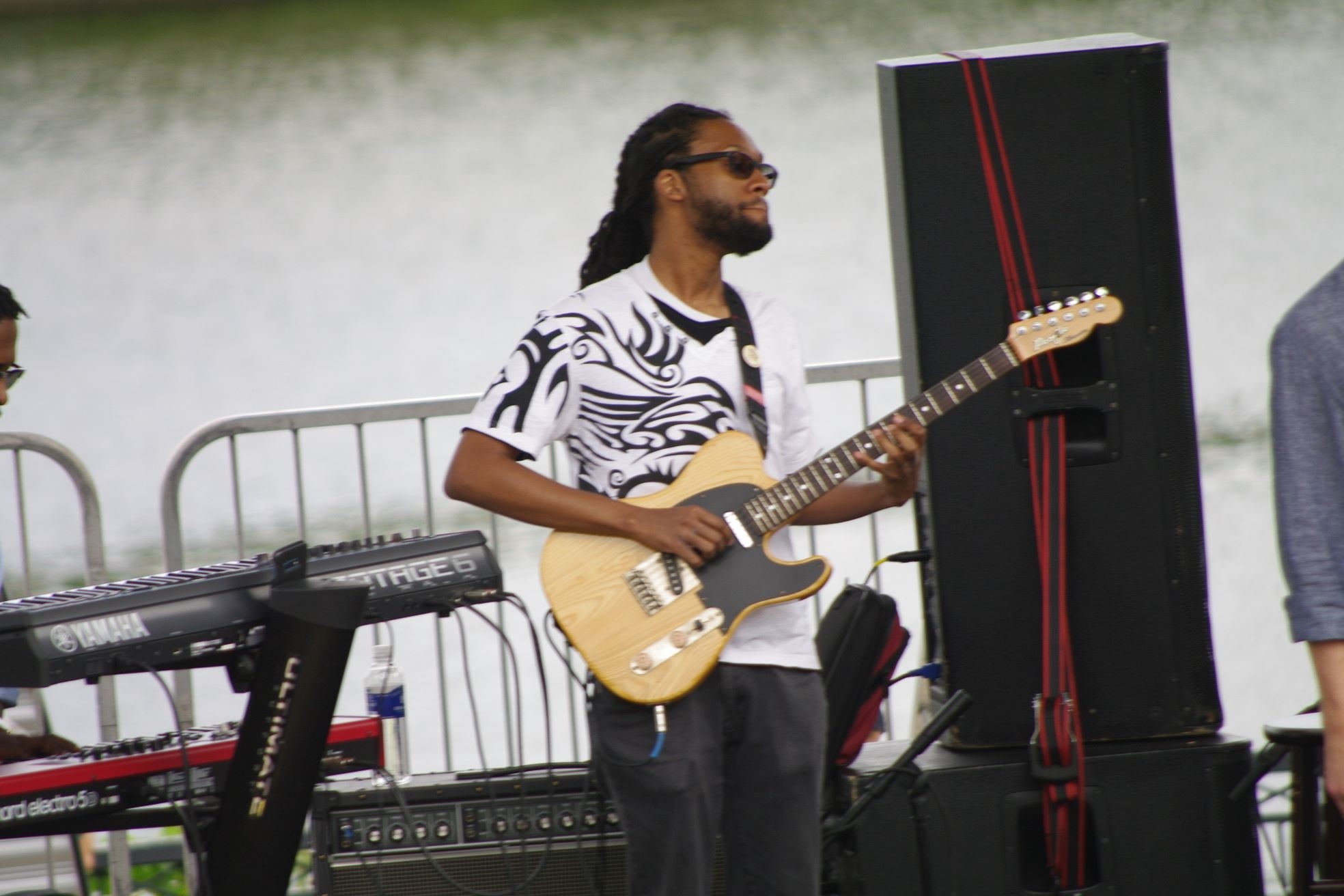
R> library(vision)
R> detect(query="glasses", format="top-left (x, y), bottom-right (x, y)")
top-left (668, 152), bottom-right (777, 191)
top-left (0, 361), bottom-right (26, 389)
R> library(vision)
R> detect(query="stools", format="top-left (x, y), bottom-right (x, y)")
top-left (1264, 713), bottom-right (1344, 896)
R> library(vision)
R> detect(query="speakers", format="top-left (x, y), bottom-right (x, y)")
top-left (874, 29), bottom-right (1224, 751)
top-left (842, 725), bottom-right (1265, 896)
top-left (311, 768), bottom-right (727, 896)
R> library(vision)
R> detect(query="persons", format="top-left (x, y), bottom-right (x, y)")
top-left (1264, 262), bottom-right (1344, 813)
top-left (0, 285), bottom-right (81, 765)
top-left (446, 103), bottom-right (930, 895)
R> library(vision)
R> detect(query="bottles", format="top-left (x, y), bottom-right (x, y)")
top-left (366, 645), bottom-right (412, 786)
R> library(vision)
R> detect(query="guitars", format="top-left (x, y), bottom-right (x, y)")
top-left (538, 286), bottom-right (1122, 707)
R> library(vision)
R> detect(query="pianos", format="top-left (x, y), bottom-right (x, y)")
top-left (1, 710), bottom-right (386, 895)
top-left (0, 527), bottom-right (504, 690)
